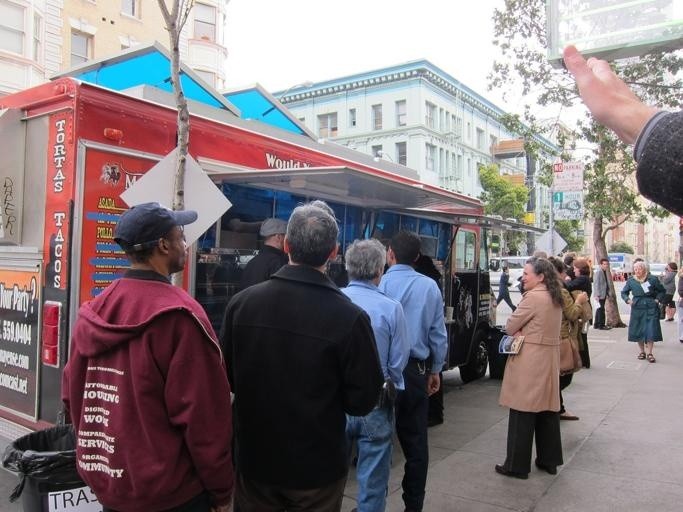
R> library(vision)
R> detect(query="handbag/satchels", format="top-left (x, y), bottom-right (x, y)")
top-left (658, 304), bottom-right (666, 320)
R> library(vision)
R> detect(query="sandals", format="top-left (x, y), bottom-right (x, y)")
top-left (638, 353), bottom-right (646, 360)
top-left (647, 354), bottom-right (655, 362)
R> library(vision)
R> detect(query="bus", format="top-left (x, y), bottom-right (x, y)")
top-left (605, 252), bottom-right (643, 280)
top-left (0, 80), bottom-right (548, 435)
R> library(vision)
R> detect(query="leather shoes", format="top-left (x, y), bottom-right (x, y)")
top-left (560, 413), bottom-right (579, 420)
top-left (495, 464), bottom-right (528, 479)
top-left (535, 457), bottom-right (557, 474)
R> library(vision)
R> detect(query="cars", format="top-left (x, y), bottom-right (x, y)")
top-left (587, 268), bottom-right (596, 282)
top-left (650, 264), bottom-right (668, 278)
top-left (592, 264), bottom-right (599, 273)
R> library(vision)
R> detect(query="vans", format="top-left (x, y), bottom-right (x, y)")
top-left (488, 255), bottom-right (532, 285)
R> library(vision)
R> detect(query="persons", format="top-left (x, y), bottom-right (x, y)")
top-left (621, 257), bottom-right (678, 362)
top-left (495, 249), bottom-right (591, 482)
top-left (61, 202), bottom-right (448, 512)
top-left (563, 44), bottom-right (683, 219)
top-left (592, 259), bottom-right (611, 330)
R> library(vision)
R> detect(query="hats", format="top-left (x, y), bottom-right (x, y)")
top-left (112, 202), bottom-right (198, 253)
top-left (259, 218), bottom-right (287, 237)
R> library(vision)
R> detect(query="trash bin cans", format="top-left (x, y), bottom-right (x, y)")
top-left (0, 423), bottom-right (103, 512)
top-left (487, 325), bottom-right (511, 379)
